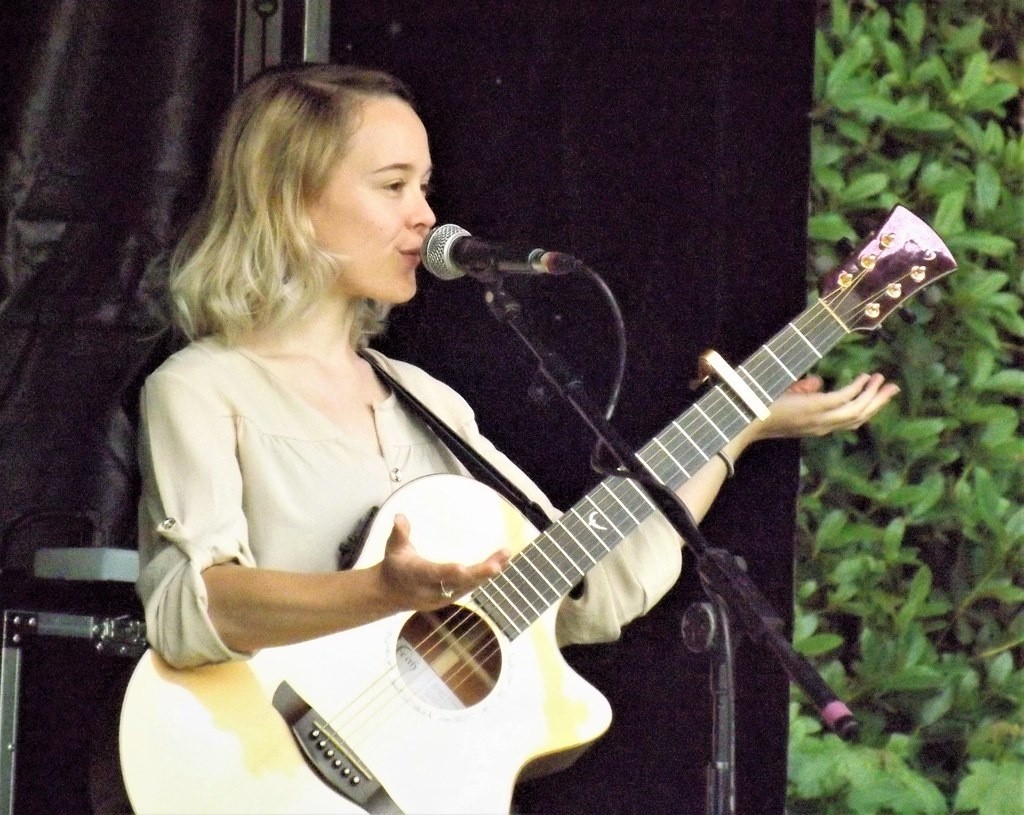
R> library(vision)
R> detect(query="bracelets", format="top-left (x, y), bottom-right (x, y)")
top-left (715, 447), bottom-right (739, 480)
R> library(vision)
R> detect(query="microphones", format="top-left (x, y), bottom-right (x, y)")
top-left (420, 223), bottom-right (583, 280)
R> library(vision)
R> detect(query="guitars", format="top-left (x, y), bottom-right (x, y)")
top-left (118, 202), bottom-right (960, 815)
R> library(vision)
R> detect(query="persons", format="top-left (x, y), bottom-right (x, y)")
top-left (133, 62), bottom-right (901, 670)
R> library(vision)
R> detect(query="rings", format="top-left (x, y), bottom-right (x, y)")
top-left (439, 578), bottom-right (455, 600)
